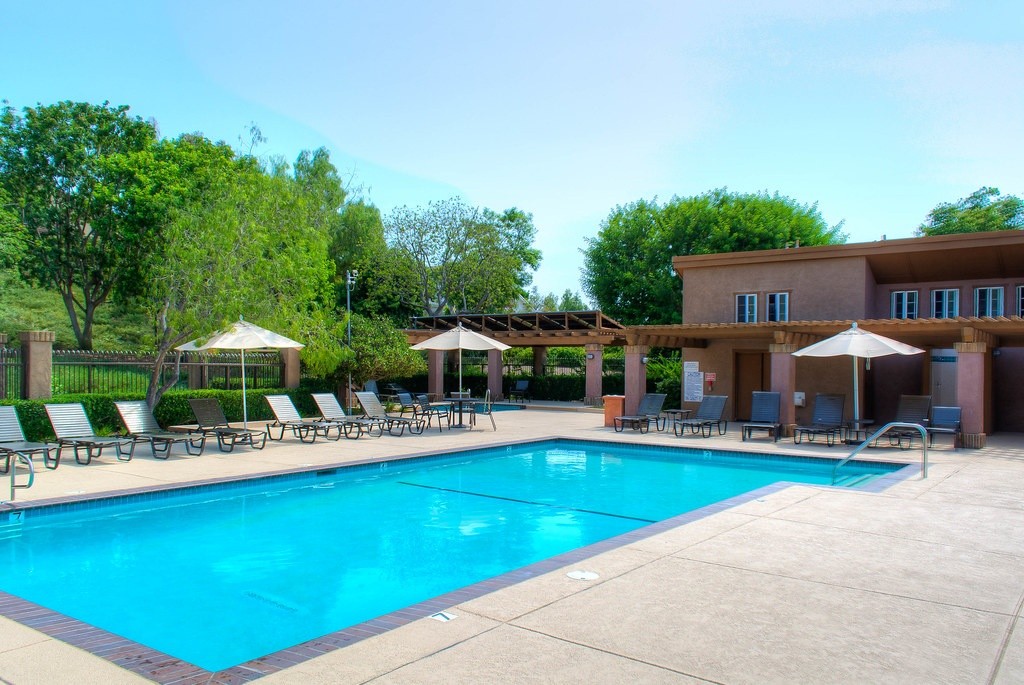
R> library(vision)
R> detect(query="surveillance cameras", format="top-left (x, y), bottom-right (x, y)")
top-left (352, 270), bottom-right (358, 276)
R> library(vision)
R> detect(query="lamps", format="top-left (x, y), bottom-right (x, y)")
top-left (587, 354), bottom-right (649, 364)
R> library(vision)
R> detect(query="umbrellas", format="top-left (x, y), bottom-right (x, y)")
top-left (174, 314), bottom-right (306, 432)
top-left (409, 322), bottom-right (512, 398)
top-left (791, 322), bottom-right (926, 430)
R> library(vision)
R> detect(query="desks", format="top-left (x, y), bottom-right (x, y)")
top-left (663, 408), bottom-right (693, 434)
top-left (844, 418), bottom-right (874, 444)
top-left (443, 397), bottom-right (479, 428)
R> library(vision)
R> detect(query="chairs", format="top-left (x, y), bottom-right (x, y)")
top-left (354, 391), bottom-right (425, 437)
top-left (114, 401), bottom-right (206, 459)
top-left (878, 394), bottom-right (964, 449)
top-left (449, 390), bottom-right (476, 425)
top-left (742, 390), bottom-right (846, 446)
top-left (508, 379), bottom-right (531, 403)
top-left (265, 394), bottom-right (343, 443)
top-left (613, 394), bottom-right (668, 433)
top-left (469, 388), bottom-right (497, 430)
top-left (189, 398), bottom-right (267, 453)
top-left (417, 394), bottom-right (450, 432)
top-left (311, 392), bottom-right (384, 440)
top-left (675, 395), bottom-right (728, 437)
top-left (397, 392), bottom-right (422, 429)
top-left (0, 405), bottom-right (61, 473)
top-left (43, 403), bottom-right (136, 465)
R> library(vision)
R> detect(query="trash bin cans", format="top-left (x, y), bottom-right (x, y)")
top-left (603, 395), bottom-right (625, 427)
